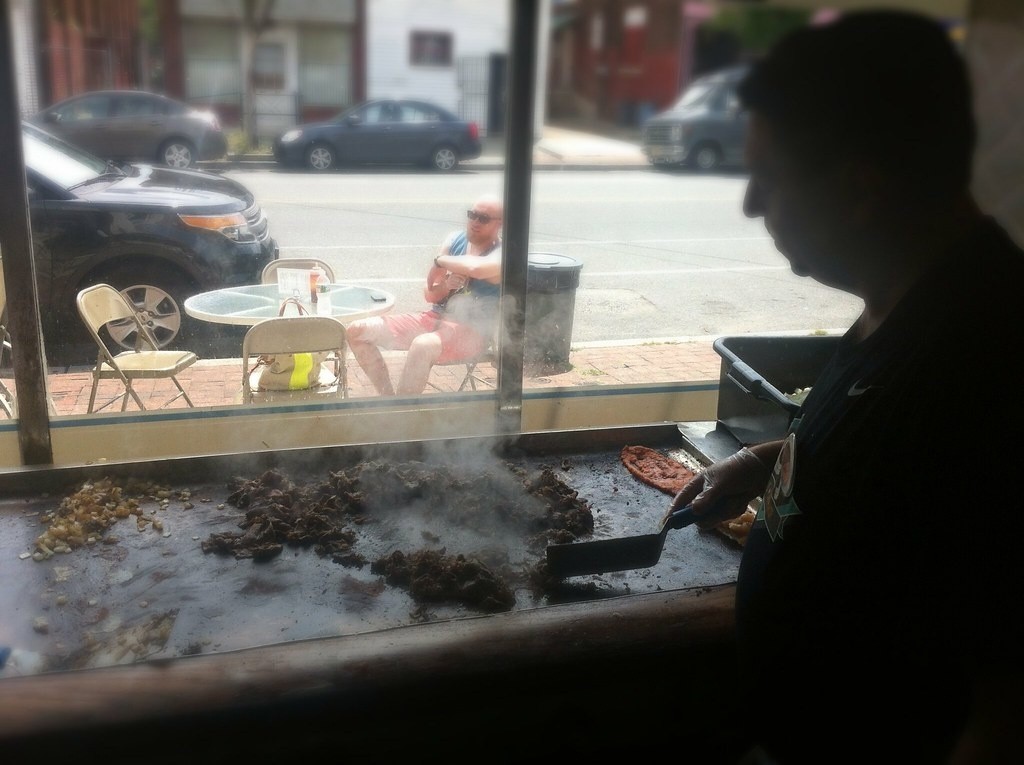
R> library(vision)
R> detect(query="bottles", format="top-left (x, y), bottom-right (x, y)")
top-left (316, 270), bottom-right (334, 317)
top-left (310, 261), bottom-right (324, 300)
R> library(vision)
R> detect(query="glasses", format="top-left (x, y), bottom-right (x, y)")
top-left (467, 210), bottom-right (501, 224)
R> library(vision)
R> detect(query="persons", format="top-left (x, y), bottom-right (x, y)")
top-left (348, 194), bottom-right (501, 398)
top-left (666, 10), bottom-right (1023, 765)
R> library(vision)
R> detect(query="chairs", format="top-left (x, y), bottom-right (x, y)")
top-left (76, 283), bottom-right (199, 414)
top-left (261, 257), bottom-right (336, 285)
top-left (455, 315), bottom-right (501, 391)
top-left (242, 317), bottom-right (349, 406)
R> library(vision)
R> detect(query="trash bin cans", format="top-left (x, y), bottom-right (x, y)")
top-left (521, 251), bottom-right (585, 372)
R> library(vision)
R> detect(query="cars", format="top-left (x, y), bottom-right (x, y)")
top-left (26, 87), bottom-right (230, 168)
top-left (271, 95), bottom-right (482, 173)
top-left (1, 120), bottom-right (280, 360)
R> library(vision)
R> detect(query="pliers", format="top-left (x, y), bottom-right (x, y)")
top-left (546, 506), bottom-right (695, 583)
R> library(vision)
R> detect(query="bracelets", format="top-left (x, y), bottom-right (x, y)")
top-left (434, 255), bottom-right (443, 267)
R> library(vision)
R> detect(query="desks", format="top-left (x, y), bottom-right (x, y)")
top-left (183, 283), bottom-right (396, 384)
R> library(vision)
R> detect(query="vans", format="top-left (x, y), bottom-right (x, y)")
top-left (636, 60), bottom-right (764, 178)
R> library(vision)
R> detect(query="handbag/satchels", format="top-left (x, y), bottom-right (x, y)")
top-left (242, 297), bottom-right (338, 391)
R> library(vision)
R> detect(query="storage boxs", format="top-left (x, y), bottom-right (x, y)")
top-left (713, 332), bottom-right (847, 450)
